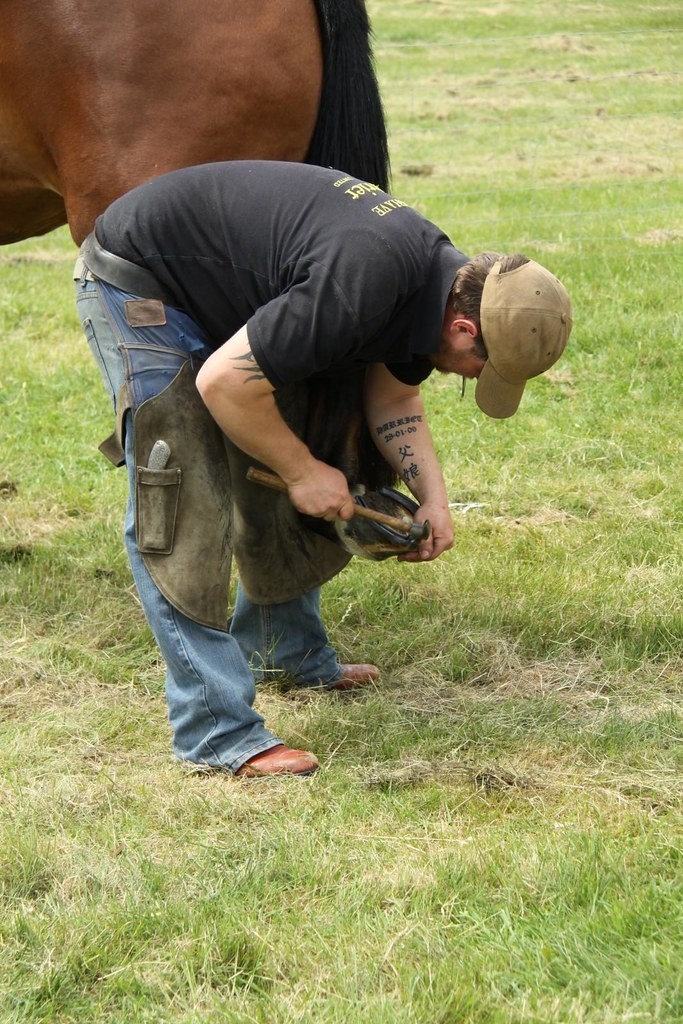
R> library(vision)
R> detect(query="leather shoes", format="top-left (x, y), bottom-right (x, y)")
top-left (326, 664), bottom-right (381, 689)
top-left (235, 743), bottom-right (319, 775)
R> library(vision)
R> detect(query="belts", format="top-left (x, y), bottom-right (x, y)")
top-left (78, 227), bottom-right (180, 306)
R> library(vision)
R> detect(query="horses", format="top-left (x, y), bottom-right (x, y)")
top-left (1, 1), bottom-right (434, 565)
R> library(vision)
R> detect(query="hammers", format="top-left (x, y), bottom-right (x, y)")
top-left (245, 465), bottom-right (432, 551)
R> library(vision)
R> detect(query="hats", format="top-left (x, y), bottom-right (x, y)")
top-left (475, 256), bottom-right (573, 418)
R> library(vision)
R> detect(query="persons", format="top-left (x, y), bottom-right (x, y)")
top-left (73, 160), bottom-right (572, 777)
